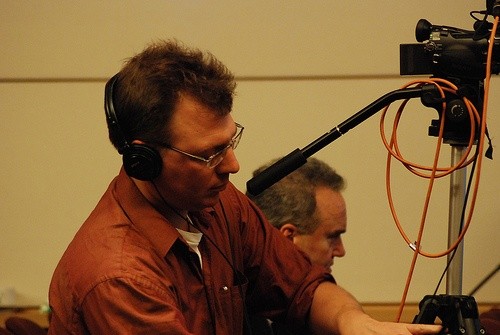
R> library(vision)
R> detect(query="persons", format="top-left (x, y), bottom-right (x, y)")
top-left (246, 154), bottom-right (349, 335)
top-left (46, 37), bottom-right (444, 335)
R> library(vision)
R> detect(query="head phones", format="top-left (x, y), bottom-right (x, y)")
top-left (105, 70), bottom-right (163, 181)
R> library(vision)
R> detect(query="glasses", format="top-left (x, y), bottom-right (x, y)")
top-left (133, 122), bottom-right (244, 169)
